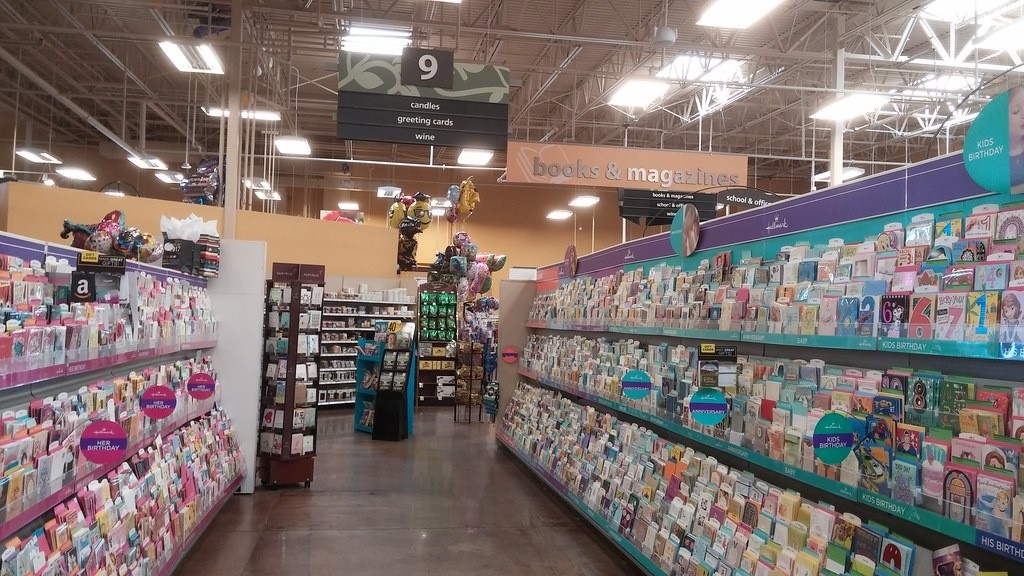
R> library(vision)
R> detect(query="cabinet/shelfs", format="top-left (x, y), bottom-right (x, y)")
top-left (318, 297), bottom-right (415, 405)
top-left (497, 324), bottom-right (1024, 574)
top-left (415, 290), bottom-right (459, 407)
top-left (374, 344), bottom-right (415, 440)
top-left (452, 333), bottom-right (491, 423)
top-left (259, 281), bottom-right (326, 487)
top-left (0, 326), bottom-right (246, 576)
top-left (354, 332), bottom-right (386, 433)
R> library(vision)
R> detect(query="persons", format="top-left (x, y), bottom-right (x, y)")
top-left (388, 333), bottom-right (397, 348)
top-left (684, 204), bottom-right (699, 256)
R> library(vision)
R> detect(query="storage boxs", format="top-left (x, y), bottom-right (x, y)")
top-left (192, 233), bottom-right (222, 278)
top-left (155, 232), bottom-right (195, 270)
top-left (257, 288), bottom-right (410, 456)
top-left (497, 201), bottom-right (1024, 576)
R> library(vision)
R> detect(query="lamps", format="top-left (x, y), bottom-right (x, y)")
top-left (13, 135), bottom-right (494, 217)
top-left (696, 0), bottom-right (780, 33)
top-left (158, 40), bottom-right (225, 75)
top-left (194, 103), bottom-right (286, 122)
top-left (546, 206), bottom-right (576, 223)
top-left (569, 188), bottom-right (599, 211)
top-left (655, 55), bottom-right (751, 84)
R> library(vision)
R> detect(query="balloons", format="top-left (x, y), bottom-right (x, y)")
top-left (388, 176), bottom-right (507, 312)
top-left (60, 209), bottom-right (165, 265)
